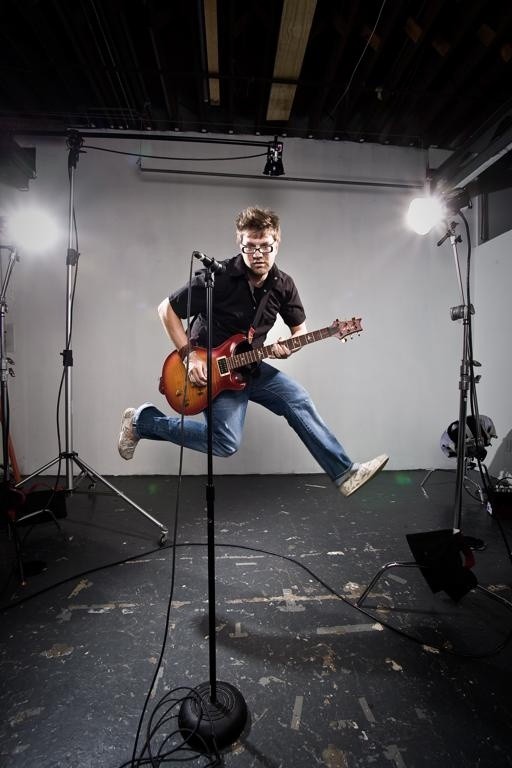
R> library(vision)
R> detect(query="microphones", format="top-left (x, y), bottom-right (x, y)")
top-left (194, 250), bottom-right (228, 275)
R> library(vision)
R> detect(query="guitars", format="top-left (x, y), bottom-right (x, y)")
top-left (160, 316), bottom-right (363, 416)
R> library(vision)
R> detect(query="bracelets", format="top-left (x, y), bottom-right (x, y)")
top-left (177, 342), bottom-right (195, 362)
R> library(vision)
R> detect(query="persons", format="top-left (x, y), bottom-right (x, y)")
top-left (117, 203), bottom-right (389, 498)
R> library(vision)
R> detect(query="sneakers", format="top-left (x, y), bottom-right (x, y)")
top-left (118, 407), bottom-right (140, 460)
top-left (338, 453), bottom-right (390, 499)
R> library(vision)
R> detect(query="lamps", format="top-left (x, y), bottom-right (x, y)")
top-left (403, 189), bottom-right (485, 548)
top-left (13, 127), bottom-right (286, 546)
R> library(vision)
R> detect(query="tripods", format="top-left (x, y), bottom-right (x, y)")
top-left (356, 230), bottom-right (512, 607)
top-left (14, 157), bottom-right (170, 544)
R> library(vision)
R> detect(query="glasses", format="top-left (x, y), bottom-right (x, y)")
top-left (240, 238), bottom-right (277, 254)
